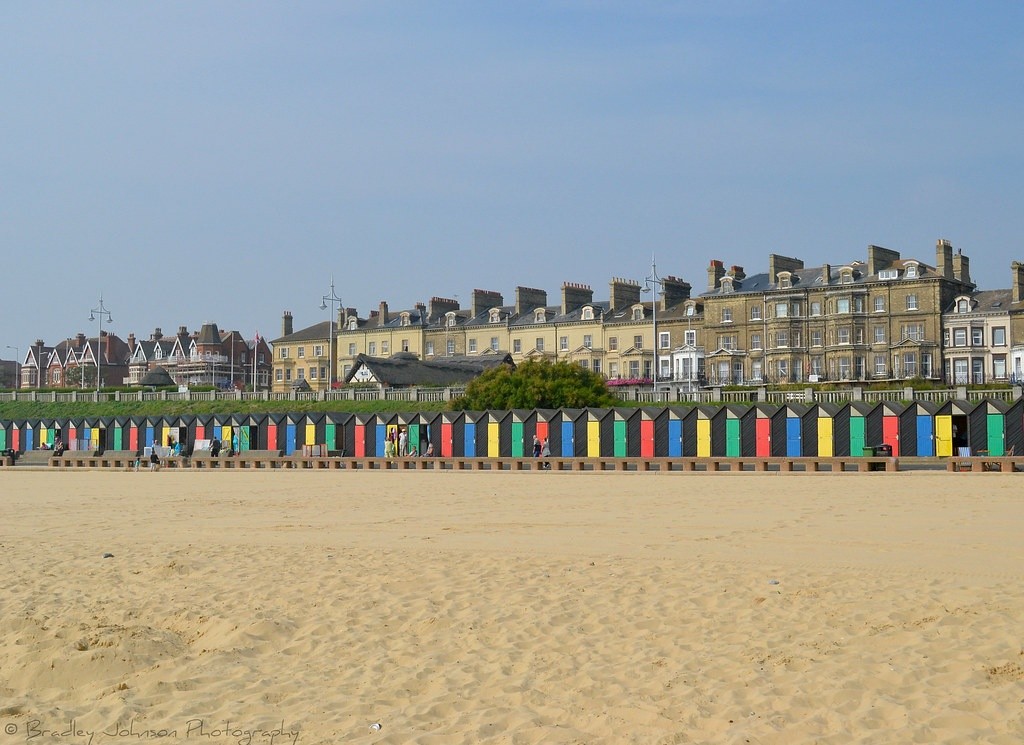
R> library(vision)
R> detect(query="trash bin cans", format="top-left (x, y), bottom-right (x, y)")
top-left (862, 447), bottom-right (874, 457)
top-left (874, 443), bottom-right (892, 471)
top-left (4, 448), bottom-right (15, 465)
top-left (0, 450), bottom-right (5, 465)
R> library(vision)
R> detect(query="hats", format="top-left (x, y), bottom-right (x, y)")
top-left (402, 429), bottom-right (405, 431)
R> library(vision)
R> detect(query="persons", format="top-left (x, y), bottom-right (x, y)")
top-left (207, 436), bottom-right (221, 465)
top-left (541, 437), bottom-right (551, 468)
top-left (165, 434), bottom-right (189, 467)
top-left (40, 435), bottom-right (64, 457)
top-left (149, 450), bottom-right (159, 472)
top-left (406, 446), bottom-right (417, 457)
top-left (953, 423), bottom-right (959, 456)
top-left (135, 458), bottom-right (141, 472)
top-left (420, 438), bottom-right (434, 457)
top-left (385, 433), bottom-right (395, 457)
top-left (399, 428), bottom-right (407, 457)
top-left (533, 435), bottom-right (541, 457)
top-left (152, 440), bottom-right (162, 451)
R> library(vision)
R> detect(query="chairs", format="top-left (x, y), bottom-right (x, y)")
top-left (958, 447), bottom-right (973, 471)
top-left (991, 445), bottom-right (1016, 471)
